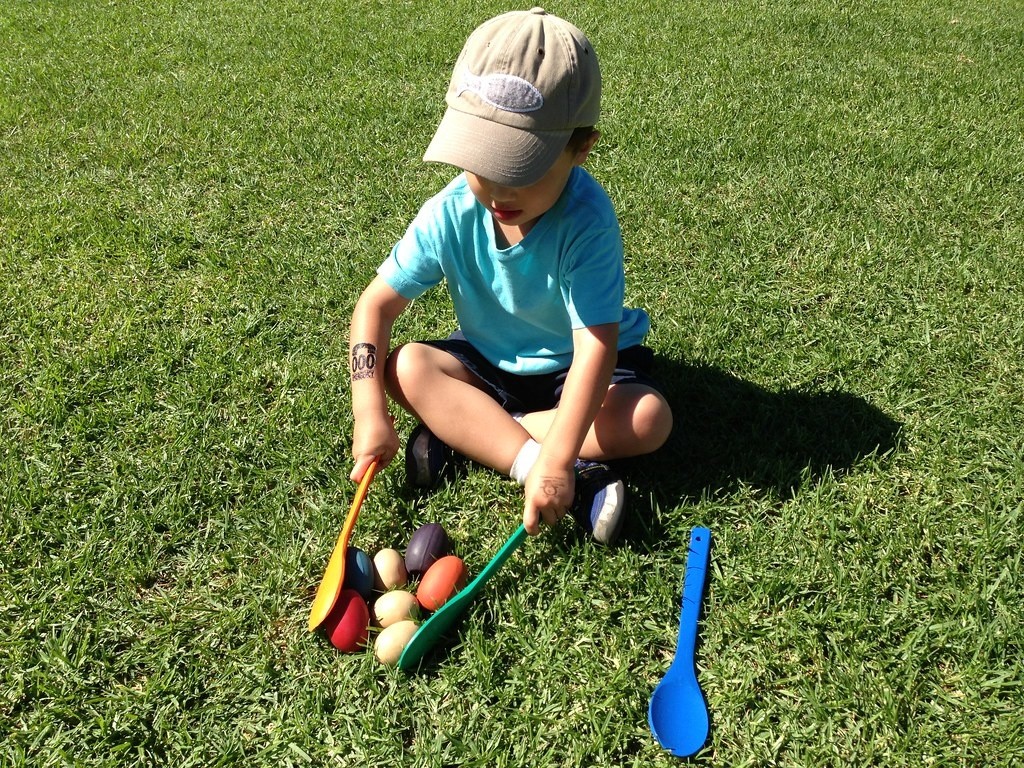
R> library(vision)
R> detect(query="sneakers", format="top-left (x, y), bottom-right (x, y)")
top-left (568, 458), bottom-right (624, 545)
top-left (405, 424), bottom-right (478, 488)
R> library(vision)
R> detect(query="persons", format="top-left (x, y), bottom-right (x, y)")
top-left (350, 7), bottom-right (675, 543)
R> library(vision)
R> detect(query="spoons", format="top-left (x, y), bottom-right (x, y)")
top-left (649, 527), bottom-right (711, 756)
top-left (398, 516), bottom-right (537, 670)
top-left (308, 456), bottom-right (381, 632)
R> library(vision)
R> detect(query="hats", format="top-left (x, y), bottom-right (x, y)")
top-left (423, 7), bottom-right (601, 189)
